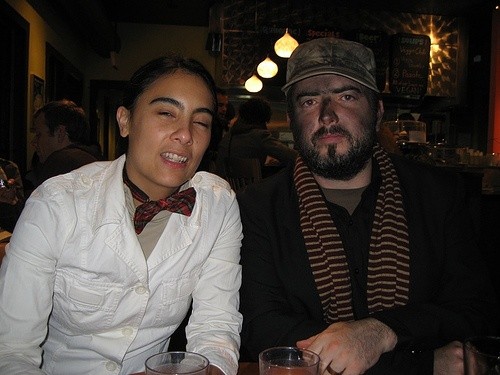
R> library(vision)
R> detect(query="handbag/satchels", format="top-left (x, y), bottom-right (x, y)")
top-left (227, 176), bottom-right (254, 194)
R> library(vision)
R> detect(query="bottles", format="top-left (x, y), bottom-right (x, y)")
top-left (462, 148), bottom-right (498, 167)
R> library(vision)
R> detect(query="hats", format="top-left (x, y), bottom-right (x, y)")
top-left (281, 38), bottom-right (391, 97)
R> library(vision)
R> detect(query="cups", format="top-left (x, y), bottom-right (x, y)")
top-left (145, 351), bottom-right (210, 375)
top-left (258, 346), bottom-right (320, 375)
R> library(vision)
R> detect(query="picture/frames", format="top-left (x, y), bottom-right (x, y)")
top-left (30, 73), bottom-right (45, 132)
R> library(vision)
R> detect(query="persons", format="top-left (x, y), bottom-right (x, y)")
top-left (30, 99), bottom-right (103, 176)
top-left (210, 97), bottom-right (298, 197)
top-left (0, 54), bottom-right (244, 375)
top-left (231, 31), bottom-right (500, 375)
top-left (194, 89), bottom-right (230, 173)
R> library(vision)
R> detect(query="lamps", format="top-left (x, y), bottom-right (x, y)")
top-left (244, 0), bottom-right (299, 93)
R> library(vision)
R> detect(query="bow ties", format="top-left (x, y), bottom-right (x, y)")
top-left (122, 167), bottom-right (197, 236)
